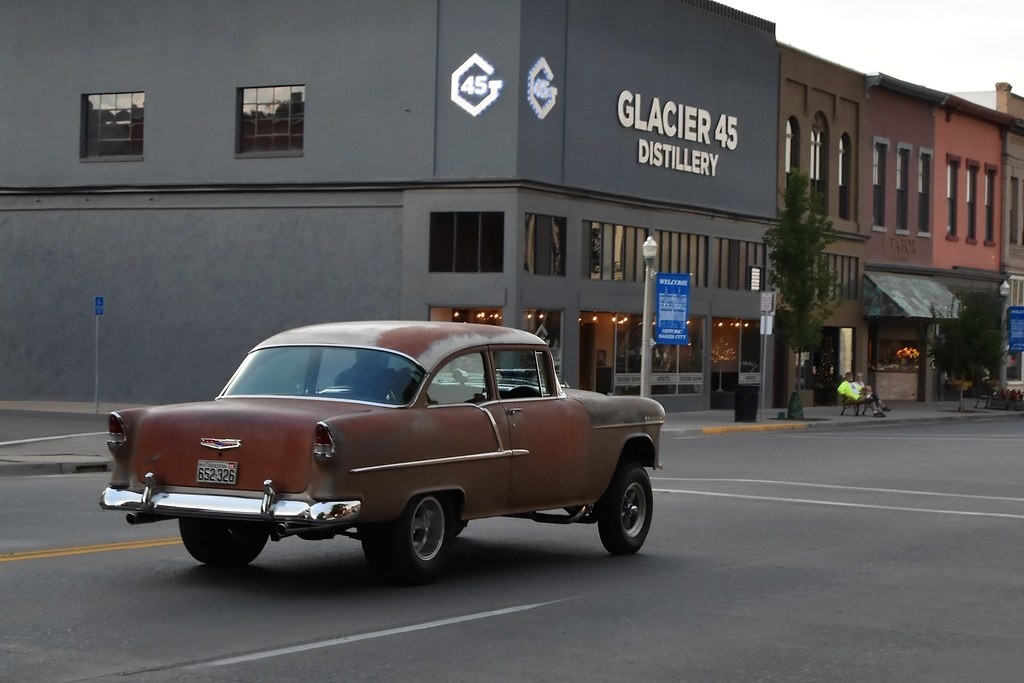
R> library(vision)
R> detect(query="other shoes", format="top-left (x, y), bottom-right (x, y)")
top-left (873, 409), bottom-right (887, 417)
top-left (881, 406), bottom-right (891, 411)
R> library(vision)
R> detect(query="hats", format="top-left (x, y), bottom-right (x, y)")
top-left (842, 371), bottom-right (852, 379)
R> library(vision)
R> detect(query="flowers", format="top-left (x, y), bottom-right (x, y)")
top-left (994, 388), bottom-right (1024, 401)
top-left (897, 348), bottom-right (919, 367)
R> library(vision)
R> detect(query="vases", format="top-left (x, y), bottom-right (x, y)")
top-left (899, 357), bottom-right (917, 369)
top-left (990, 400), bottom-right (1024, 411)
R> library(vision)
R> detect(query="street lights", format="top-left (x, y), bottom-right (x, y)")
top-left (639, 236), bottom-right (659, 397)
top-left (999, 280), bottom-right (1010, 396)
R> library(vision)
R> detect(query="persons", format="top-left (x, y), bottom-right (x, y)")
top-left (333, 349), bottom-right (439, 406)
top-left (982, 366), bottom-right (990, 395)
top-left (838, 372), bottom-right (891, 417)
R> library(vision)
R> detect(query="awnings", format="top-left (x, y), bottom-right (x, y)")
top-left (863, 273), bottom-right (971, 318)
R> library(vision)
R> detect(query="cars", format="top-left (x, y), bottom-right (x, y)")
top-left (98, 319), bottom-right (667, 586)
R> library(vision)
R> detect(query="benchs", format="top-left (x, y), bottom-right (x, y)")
top-left (840, 396), bottom-right (875, 416)
top-left (974, 383), bottom-right (997, 409)
